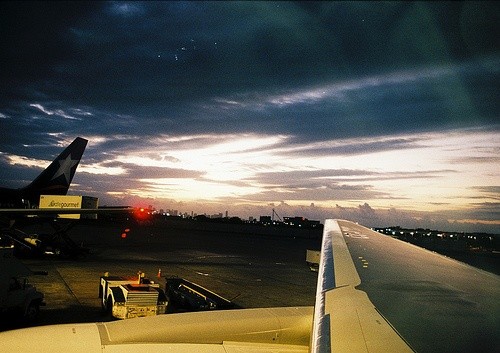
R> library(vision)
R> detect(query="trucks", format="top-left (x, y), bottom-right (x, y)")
top-left (98, 270), bottom-right (169, 318)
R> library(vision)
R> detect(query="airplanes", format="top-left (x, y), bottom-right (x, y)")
top-left (0, 136), bottom-right (152, 265)
